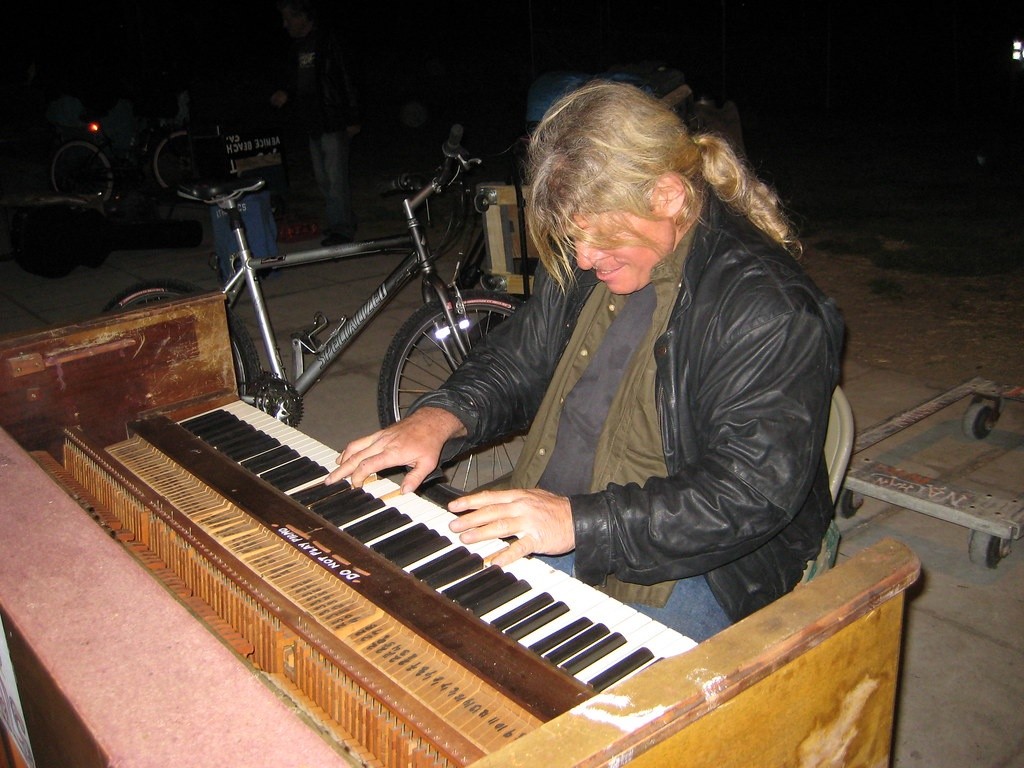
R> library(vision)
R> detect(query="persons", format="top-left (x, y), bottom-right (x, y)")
top-left (324, 80), bottom-right (847, 645)
top-left (271, 0), bottom-right (365, 246)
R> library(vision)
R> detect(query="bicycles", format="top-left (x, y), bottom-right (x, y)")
top-left (51, 106), bottom-right (207, 206)
top-left (102, 123), bottom-right (532, 512)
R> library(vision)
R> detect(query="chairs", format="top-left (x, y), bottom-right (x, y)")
top-left (795, 378), bottom-right (858, 583)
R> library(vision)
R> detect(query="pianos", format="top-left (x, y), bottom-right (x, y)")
top-left (2, 289), bottom-right (923, 766)
